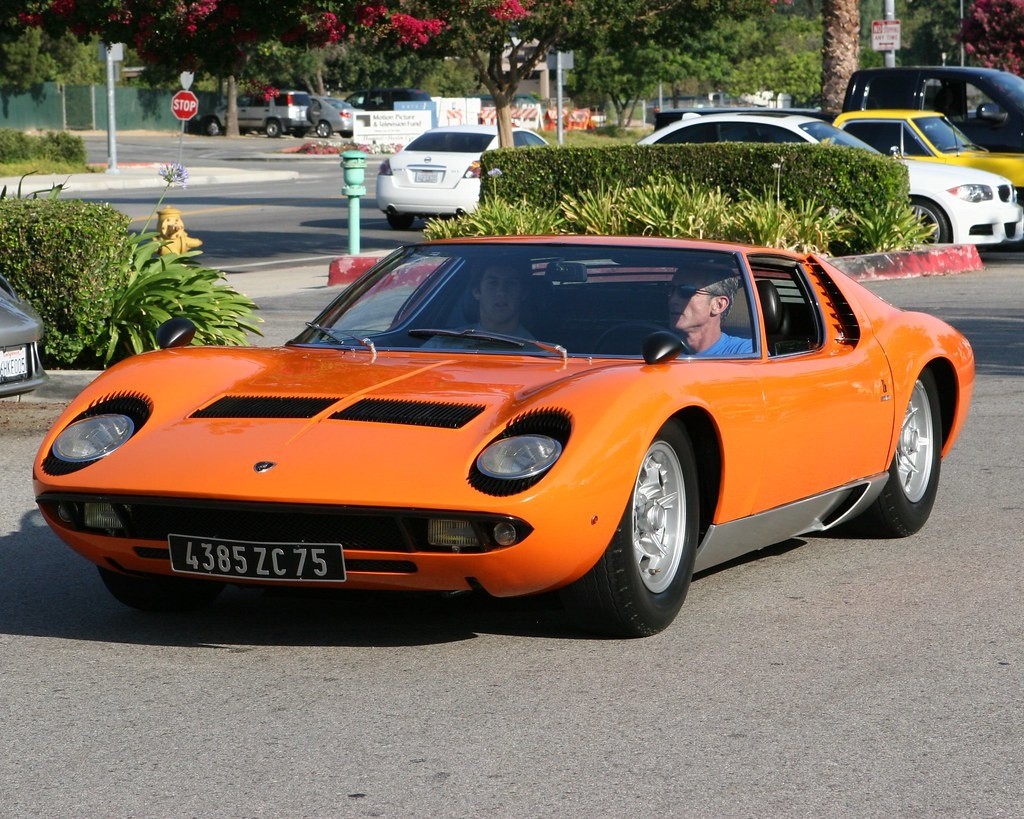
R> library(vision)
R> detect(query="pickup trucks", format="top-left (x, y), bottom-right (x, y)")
top-left (656, 63), bottom-right (1023, 154)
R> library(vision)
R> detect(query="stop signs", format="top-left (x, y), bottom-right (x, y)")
top-left (171, 89), bottom-right (198, 122)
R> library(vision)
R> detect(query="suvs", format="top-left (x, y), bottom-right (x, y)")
top-left (344, 88), bottom-right (439, 128)
top-left (201, 90), bottom-right (321, 139)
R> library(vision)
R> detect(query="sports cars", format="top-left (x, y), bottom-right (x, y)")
top-left (35, 235), bottom-right (977, 640)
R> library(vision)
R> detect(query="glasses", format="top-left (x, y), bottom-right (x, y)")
top-left (664, 283), bottom-right (720, 298)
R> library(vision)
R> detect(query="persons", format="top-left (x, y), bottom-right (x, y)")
top-left (616, 265), bottom-right (771, 357)
top-left (419, 258), bottom-right (540, 354)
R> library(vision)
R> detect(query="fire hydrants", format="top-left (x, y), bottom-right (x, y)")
top-left (152, 206), bottom-right (202, 255)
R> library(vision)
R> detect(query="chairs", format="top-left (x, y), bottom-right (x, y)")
top-left (721, 279), bottom-right (792, 345)
top-left (462, 275), bottom-right (554, 342)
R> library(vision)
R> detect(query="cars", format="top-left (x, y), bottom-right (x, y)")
top-left (630, 113), bottom-right (1024, 255)
top-left (813, 108), bottom-right (1024, 193)
top-left (309, 97), bottom-right (366, 138)
top-left (375, 126), bottom-right (553, 229)
top-left (0, 274), bottom-right (50, 398)
